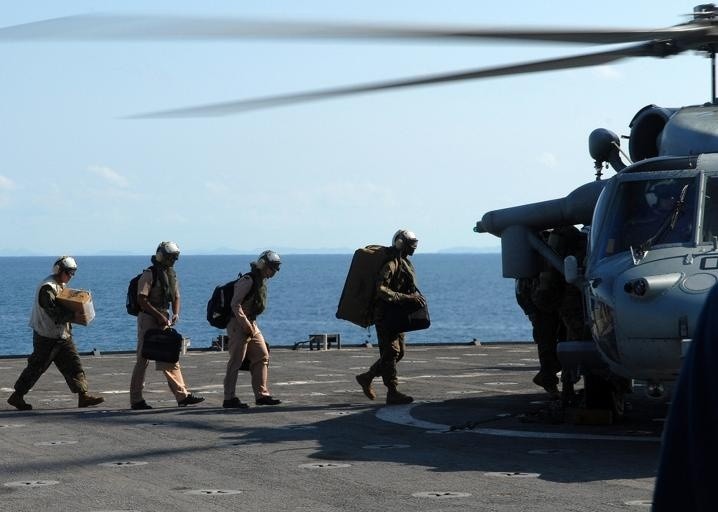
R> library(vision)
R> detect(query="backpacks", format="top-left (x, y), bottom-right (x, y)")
top-left (126, 266), bottom-right (156, 317)
top-left (336, 245), bottom-right (399, 329)
top-left (206, 273), bottom-right (259, 329)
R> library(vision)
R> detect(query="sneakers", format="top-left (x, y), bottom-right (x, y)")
top-left (534, 371), bottom-right (558, 392)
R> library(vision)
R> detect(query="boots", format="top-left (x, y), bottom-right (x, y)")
top-left (387, 388), bottom-right (413, 404)
top-left (76, 391), bottom-right (105, 408)
top-left (6, 390), bottom-right (32, 410)
top-left (357, 371), bottom-right (376, 399)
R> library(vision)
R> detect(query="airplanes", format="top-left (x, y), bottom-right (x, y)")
top-left (0, 3), bottom-right (718, 430)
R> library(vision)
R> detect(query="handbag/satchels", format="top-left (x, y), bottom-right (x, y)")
top-left (240, 342), bottom-right (270, 372)
top-left (141, 329), bottom-right (182, 363)
top-left (388, 296), bottom-right (430, 331)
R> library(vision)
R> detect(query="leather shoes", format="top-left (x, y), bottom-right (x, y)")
top-left (130, 400), bottom-right (151, 409)
top-left (256, 396), bottom-right (281, 405)
top-left (179, 393), bottom-right (206, 407)
top-left (223, 397), bottom-right (250, 409)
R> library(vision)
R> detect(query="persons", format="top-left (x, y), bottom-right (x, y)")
top-left (355, 230), bottom-right (425, 406)
top-left (509, 223), bottom-right (592, 404)
top-left (128, 242), bottom-right (206, 411)
top-left (222, 250), bottom-right (280, 409)
top-left (7, 255), bottom-right (105, 411)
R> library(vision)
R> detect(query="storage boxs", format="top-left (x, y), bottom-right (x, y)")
top-left (56, 288), bottom-right (96, 326)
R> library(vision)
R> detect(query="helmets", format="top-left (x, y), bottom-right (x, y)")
top-left (392, 229), bottom-right (418, 256)
top-left (256, 250), bottom-right (280, 277)
top-left (155, 242), bottom-right (180, 263)
top-left (52, 257), bottom-right (78, 275)
